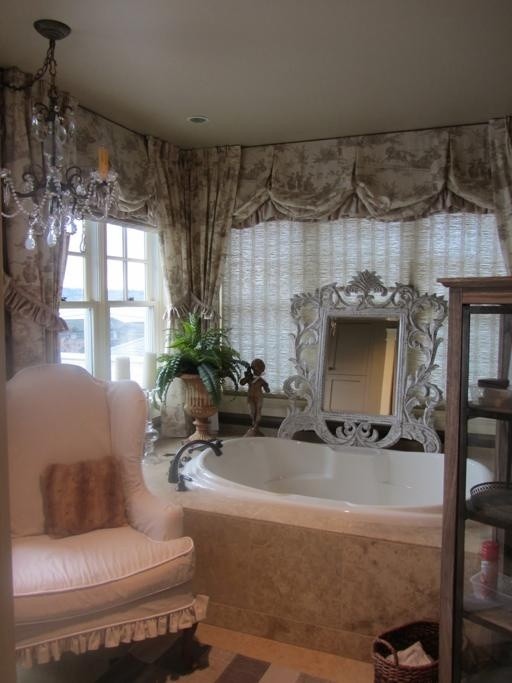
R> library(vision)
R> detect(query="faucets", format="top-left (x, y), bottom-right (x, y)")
top-left (168, 439), bottom-right (223, 484)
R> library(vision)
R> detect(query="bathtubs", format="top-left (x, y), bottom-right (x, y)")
top-left (182, 437), bottom-right (494, 523)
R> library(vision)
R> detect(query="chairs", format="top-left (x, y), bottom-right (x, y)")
top-left (5, 360), bottom-right (210, 672)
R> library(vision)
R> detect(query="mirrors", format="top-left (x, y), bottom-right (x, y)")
top-left (275, 268), bottom-right (442, 453)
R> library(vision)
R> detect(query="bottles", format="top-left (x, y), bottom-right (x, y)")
top-left (479, 540), bottom-right (499, 597)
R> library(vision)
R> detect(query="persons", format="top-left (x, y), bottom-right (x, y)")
top-left (240, 358), bottom-right (270, 431)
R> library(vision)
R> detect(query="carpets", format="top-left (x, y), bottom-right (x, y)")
top-left (95, 641), bottom-right (338, 681)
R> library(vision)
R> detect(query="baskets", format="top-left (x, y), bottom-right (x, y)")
top-left (371, 618), bottom-right (467, 683)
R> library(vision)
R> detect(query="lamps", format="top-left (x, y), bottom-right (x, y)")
top-left (0, 19), bottom-right (118, 248)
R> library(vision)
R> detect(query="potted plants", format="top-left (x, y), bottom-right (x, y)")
top-left (155, 313), bottom-right (250, 445)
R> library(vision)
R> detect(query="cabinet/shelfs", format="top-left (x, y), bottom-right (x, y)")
top-left (435, 277), bottom-right (512, 683)
